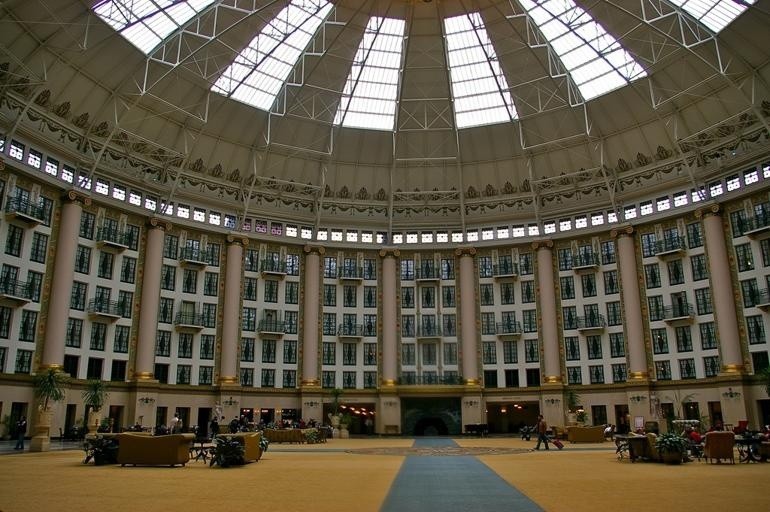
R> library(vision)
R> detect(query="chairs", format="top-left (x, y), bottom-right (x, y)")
top-left (759, 438), bottom-right (770, 462)
top-left (604, 425), bottom-right (615, 442)
top-left (702, 431), bottom-right (735, 465)
top-left (550, 425), bottom-right (566, 442)
top-left (216, 432), bottom-right (262, 463)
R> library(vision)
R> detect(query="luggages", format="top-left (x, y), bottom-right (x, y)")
top-left (545, 434), bottom-right (564, 450)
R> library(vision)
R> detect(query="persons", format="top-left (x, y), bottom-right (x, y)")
top-left (13, 414), bottom-right (27, 452)
top-left (59, 412), bottom-right (326, 434)
top-left (530, 414), bottom-right (549, 450)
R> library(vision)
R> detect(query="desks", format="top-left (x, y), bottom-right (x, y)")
top-left (616, 436), bottom-right (648, 463)
top-left (734, 437), bottom-right (763, 464)
top-left (188, 446), bottom-right (215, 463)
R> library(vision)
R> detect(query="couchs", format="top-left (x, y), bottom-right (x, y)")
top-left (85, 432), bottom-right (155, 445)
top-left (117, 433), bottom-right (196, 467)
top-left (565, 423), bottom-right (605, 444)
top-left (628, 431), bottom-right (656, 461)
top-left (263, 427), bottom-right (320, 444)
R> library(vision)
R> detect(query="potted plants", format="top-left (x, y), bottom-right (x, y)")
top-left (576, 412), bottom-right (587, 427)
top-left (209, 436), bottom-right (243, 467)
top-left (654, 432), bottom-right (689, 465)
top-left (341, 412), bottom-right (352, 439)
top-left (565, 386), bottom-right (581, 426)
top-left (29, 368), bottom-right (72, 452)
top-left (327, 388), bottom-right (340, 438)
top-left (81, 376), bottom-right (110, 436)
